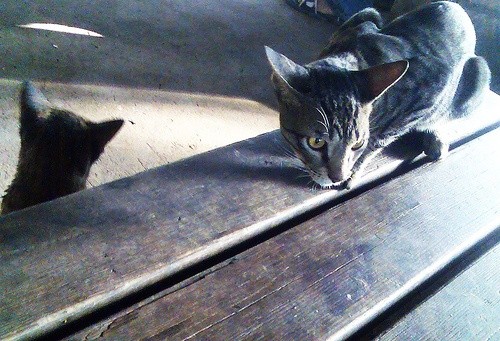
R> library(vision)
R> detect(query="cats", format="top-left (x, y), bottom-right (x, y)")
top-left (263, 0), bottom-right (492, 191)
top-left (0, 79), bottom-right (125, 216)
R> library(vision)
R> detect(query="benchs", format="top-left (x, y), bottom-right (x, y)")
top-left (0, 90), bottom-right (498, 337)
